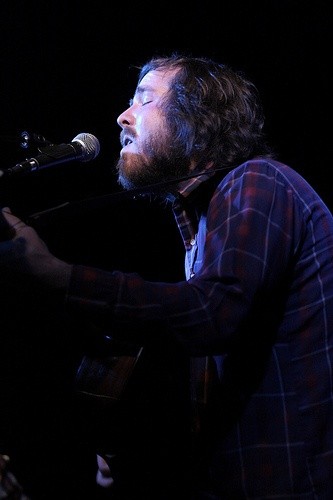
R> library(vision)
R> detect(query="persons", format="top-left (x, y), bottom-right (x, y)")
top-left (0, 54), bottom-right (332, 500)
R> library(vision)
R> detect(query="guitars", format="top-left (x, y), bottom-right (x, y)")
top-left (0, 212), bottom-right (220, 464)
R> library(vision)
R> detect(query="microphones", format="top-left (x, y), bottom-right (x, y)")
top-left (7, 132), bottom-right (100, 175)
